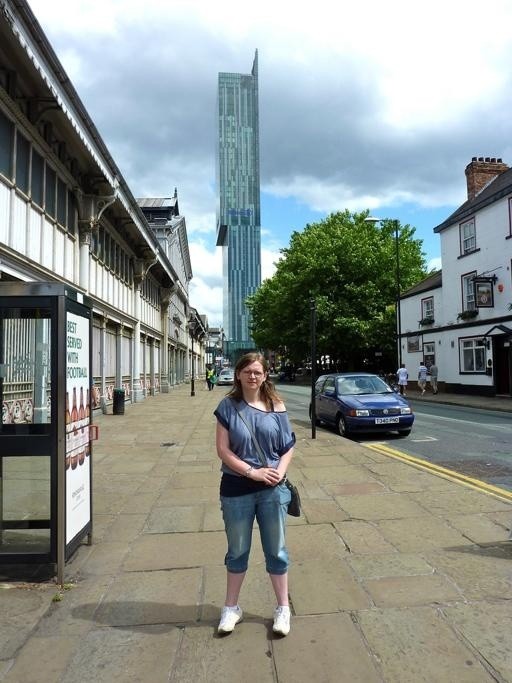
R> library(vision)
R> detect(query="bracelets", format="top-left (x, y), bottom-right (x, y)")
top-left (246, 466), bottom-right (253, 477)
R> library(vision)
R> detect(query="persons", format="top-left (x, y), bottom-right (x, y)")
top-left (397, 364), bottom-right (408, 396)
top-left (427, 361), bottom-right (438, 394)
top-left (206, 364), bottom-right (216, 391)
top-left (214, 353), bottom-right (297, 636)
top-left (417, 362), bottom-right (428, 395)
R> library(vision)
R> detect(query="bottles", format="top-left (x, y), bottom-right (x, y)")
top-left (65, 385), bottom-right (90, 470)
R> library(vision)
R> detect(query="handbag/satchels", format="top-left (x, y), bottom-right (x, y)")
top-left (285, 480), bottom-right (301, 517)
top-left (209, 375), bottom-right (217, 384)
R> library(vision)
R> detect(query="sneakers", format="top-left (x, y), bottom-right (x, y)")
top-left (218, 604), bottom-right (242, 634)
top-left (272, 606), bottom-right (291, 636)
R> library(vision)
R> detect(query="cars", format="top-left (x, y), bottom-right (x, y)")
top-left (216, 366), bottom-right (235, 386)
top-left (308, 372), bottom-right (417, 441)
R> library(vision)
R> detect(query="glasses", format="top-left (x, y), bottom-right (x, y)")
top-left (244, 370), bottom-right (263, 377)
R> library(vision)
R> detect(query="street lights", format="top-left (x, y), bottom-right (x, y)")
top-left (184, 323), bottom-right (195, 395)
top-left (362, 217), bottom-right (405, 395)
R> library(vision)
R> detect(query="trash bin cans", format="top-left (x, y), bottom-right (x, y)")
top-left (113, 389), bottom-right (125, 415)
